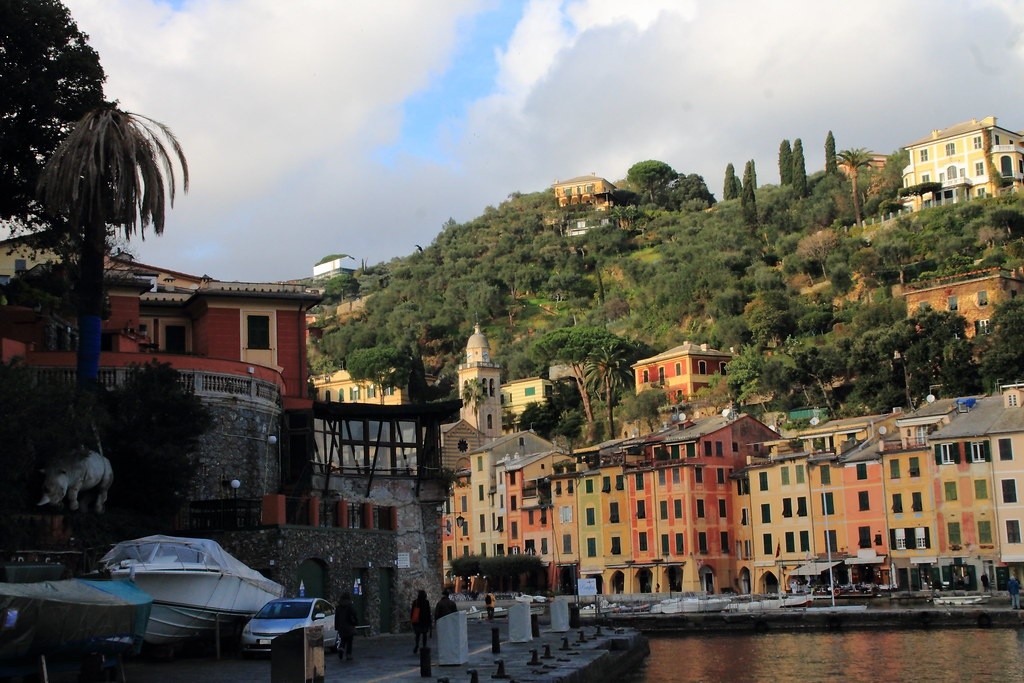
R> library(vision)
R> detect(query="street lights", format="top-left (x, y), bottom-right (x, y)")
top-left (816, 483), bottom-right (836, 607)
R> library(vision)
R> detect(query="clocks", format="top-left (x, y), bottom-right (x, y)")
top-left (482, 351), bottom-right (489, 360)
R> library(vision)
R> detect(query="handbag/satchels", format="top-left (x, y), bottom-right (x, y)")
top-left (411, 600), bottom-right (420, 623)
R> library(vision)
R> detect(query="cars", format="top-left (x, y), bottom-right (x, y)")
top-left (238, 597), bottom-right (345, 659)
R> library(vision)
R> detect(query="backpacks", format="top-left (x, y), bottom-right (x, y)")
top-left (486, 595), bottom-right (493, 605)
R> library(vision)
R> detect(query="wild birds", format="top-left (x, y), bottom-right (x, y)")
top-left (414, 245), bottom-right (423, 252)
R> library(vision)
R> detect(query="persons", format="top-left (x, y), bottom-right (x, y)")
top-left (948, 571), bottom-right (959, 590)
top-left (981, 571), bottom-right (989, 592)
top-left (833, 581), bottom-right (839, 590)
top-left (708, 582), bottom-right (713, 594)
top-left (1008, 571), bottom-right (1022, 610)
top-left (675, 583), bottom-right (681, 592)
top-left (410, 589), bottom-right (432, 654)
top-left (932, 576), bottom-right (942, 599)
top-left (964, 572), bottom-right (970, 591)
top-left (334, 593), bottom-right (355, 660)
top-left (786, 579), bottom-right (796, 594)
top-left (645, 582), bottom-right (660, 592)
top-left (434, 591), bottom-right (458, 622)
top-left (803, 576), bottom-right (822, 593)
top-left (619, 582), bottom-right (624, 594)
top-left (464, 590), bottom-right (479, 601)
top-left (485, 591), bottom-right (495, 620)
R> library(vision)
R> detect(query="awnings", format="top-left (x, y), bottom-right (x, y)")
top-left (789, 561), bottom-right (842, 575)
top-left (844, 556), bottom-right (887, 564)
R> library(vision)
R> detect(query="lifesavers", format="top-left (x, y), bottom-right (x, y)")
top-left (919, 611), bottom-right (932, 623)
top-left (755, 621), bottom-right (768, 633)
top-left (834, 588), bottom-right (840, 595)
top-left (828, 616), bottom-right (841, 627)
top-left (978, 614), bottom-right (991, 628)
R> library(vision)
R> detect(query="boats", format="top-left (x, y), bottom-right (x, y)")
top-left (99, 535), bottom-right (288, 648)
top-left (481, 606), bottom-right (508, 618)
top-left (580, 594), bottom-right (621, 614)
top-left (624, 590), bottom-right (815, 613)
top-left (465, 604), bottom-right (482, 619)
top-left (0, 564), bottom-right (154, 659)
top-left (932, 594), bottom-right (992, 607)
top-left (514, 593), bottom-right (548, 603)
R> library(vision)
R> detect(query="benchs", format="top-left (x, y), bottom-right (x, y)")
top-left (356, 625), bottom-right (371, 637)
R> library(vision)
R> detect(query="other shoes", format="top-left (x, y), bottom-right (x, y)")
top-left (339, 649), bottom-right (344, 661)
top-left (345, 655), bottom-right (354, 660)
top-left (413, 646), bottom-right (419, 654)
